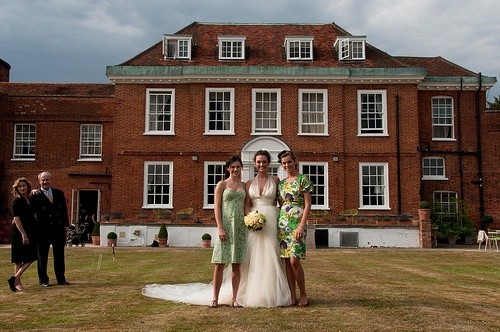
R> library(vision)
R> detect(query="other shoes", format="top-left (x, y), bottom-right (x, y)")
top-left (59, 280), bottom-right (70, 285)
top-left (232, 300), bottom-right (242, 307)
top-left (297, 301), bottom-right (308, 307)
top-left (210, 300), bottom-right (218, 307)
top-left (8, 276), bottom-right (17, 292)
top-left (42, 283), bottom-right (51, 287)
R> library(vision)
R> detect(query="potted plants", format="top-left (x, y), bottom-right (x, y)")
top-left (135, 214), bottom-right (148, 222)
top-left (417, 201), bottom-right (431, 219)
top-left (203, 216), bottom-right (216, 224)
top-left (158, 223), bottom-right (168, 246)
top-left (92, 221), bottom-right (100, 245)
top-left (107, 232), bottom-right (117, 247)
top-left (110, 213), bottom-right (126, 223)
top-left (431, 214), bottom-right (441, 229)
top-left (375, 215), bottom-right (391, 225)
top-left (202, 233), bottom-right (212, 246)
top-left (442, 222), bottom-right (474, 245)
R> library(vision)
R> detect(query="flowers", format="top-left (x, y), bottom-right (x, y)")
top-left (153, 208), bottom-right (172, 219)
top-left (243, 210), bottom-right (267, 232)
top-left (397, 214), bottom-right (410, 220)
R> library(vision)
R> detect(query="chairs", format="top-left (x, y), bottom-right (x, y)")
top-left (177, 207), bottom-right (193, 221)
top-left (477, 228), bottom-right (500, 251)
top-left (339, 209), bottom-right (358, 224)
top-left (310, 210), bottom-right (329, 224)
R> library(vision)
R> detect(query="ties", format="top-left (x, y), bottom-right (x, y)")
top-left (46, 191), bottom-right (52, 202)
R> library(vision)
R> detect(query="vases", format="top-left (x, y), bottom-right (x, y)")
top-left (158, 219), bottom-right (172, 223)
top-left (398, 220), bottom-right (411, 225)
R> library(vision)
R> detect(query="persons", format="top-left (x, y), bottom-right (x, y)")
top-left (211, 155), bottom-right (246, 308)
top-left (30, 171), bottom-right (70, 287)
top-left (141, 149), bottom-right (291, 308)
top-left (6, 177), bottom-right (41, 292)
top-left (277, 150), bottom-right (314, 306)
